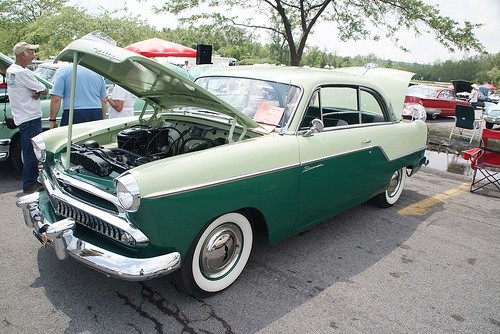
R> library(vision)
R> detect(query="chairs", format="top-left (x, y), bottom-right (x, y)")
top-left (462, 129), bottom-right (500, 192)
top-left (447, 104), bottom-right (482, 143)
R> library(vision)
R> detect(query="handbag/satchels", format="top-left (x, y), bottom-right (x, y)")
top-left (3, 116), bottom-right (17, 130)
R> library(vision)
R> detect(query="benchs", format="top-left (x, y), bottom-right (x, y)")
top-left (302, 116), bottom-right (348, 127)
top-left (309, 105), bottom-right (374, 124)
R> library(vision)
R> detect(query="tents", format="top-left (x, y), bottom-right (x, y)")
top-left (123, 37), bottom-right (197, 57)
top-left (479, 83), bottom-right (497, 90)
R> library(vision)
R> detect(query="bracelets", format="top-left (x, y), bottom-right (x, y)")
top-left (48, 119), bottom-right (56, 121)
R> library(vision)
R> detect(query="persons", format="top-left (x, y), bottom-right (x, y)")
top-left (183, 61), bottom-right (192, 71)
top-left (105, 83), bottom-right (136, 118)
top-left (49, 62), bottom-right (107, 129)
top-left (6, 42), bottom-right (49, 193)
top-left (468, 84), bottom-right (480, 109)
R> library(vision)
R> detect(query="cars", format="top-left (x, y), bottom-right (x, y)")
top-left (14, 38), bottom-right (430, 299)
top-left (456, 90), bottom-right (500, 129)
top-left (0, 48), bottom-right (194, 179)
top-left (403, 84), bottom-right (471, 120)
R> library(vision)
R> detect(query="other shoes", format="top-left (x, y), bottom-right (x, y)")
top-left (23, 181), bottom-right (44, 193)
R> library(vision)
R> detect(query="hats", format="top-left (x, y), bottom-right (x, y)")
top-left (471, 84), bottom-right (479, 90)
top-left (13, 42), bottom-right (39, 55)
